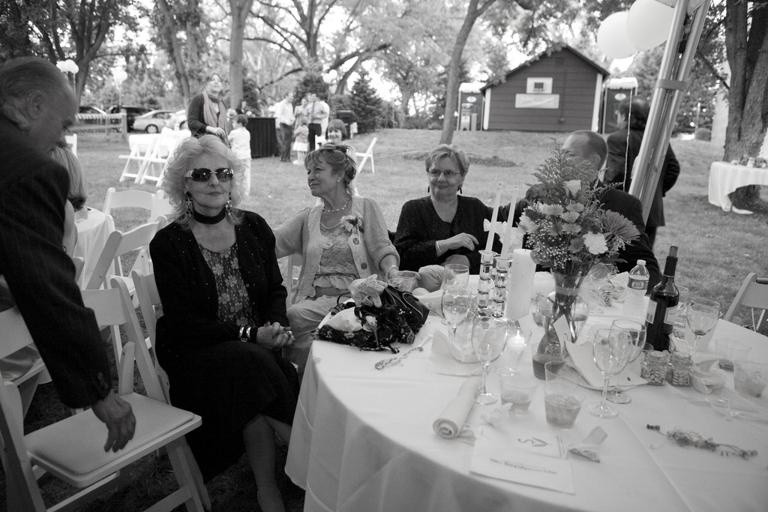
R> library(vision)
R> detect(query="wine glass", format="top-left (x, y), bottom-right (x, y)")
top-left (685, 297), bottom-right (720, 362)
top-left (443, 264), bottom-right (469, 294)
top-left (441, 289), bottom-right (472, 339)
top-left (590, 329), bottom-right (631, 417)
top-left (679, 287), bottom-right (690, 311)
top-left (471, 315), bottom-right (508, 405)
top-left (604, 320), bottom-right (646, 403)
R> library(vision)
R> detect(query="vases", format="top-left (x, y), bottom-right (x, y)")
top-left (532, 272), bottom-right (584, 366)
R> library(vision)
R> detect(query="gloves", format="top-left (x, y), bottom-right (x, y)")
top-left (346, 272), bottom-right (388, 306)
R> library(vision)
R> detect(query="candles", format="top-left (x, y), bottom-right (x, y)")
top-left (485, 188), bottom-right (503, 253)
top-left (503, 188), bottom-right (519, 255)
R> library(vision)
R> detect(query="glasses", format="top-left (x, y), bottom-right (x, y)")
top-left (184, 168), bottom-right (237, 182)
top-left (428, 169), bottom-right (462, 178)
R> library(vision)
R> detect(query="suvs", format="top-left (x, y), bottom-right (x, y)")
top-left (108, 105), bottom-right (157, 133)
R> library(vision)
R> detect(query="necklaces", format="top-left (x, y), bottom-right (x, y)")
top-left (187, 207), bottom-right (228, 225)
top-left (319, 197), bottom-right (349, 213)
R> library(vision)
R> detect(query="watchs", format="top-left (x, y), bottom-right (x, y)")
top-left (240, 324), bottom-right (257, 343)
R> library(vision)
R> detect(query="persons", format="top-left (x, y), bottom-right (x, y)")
top-left (148, 134), bottom-right (304, 512)
top-left (606, 96), bottom-right (680, 254)
top-left (271, 142), bottom-right (402, 367)
top-left (391, 141), bottom-right (504, 273)
top-left (227, 115), bottom-right (251, 209)
top-left (314, 118), bottom-right (355, 201)
top-left (188, 71), bottom-right (232, 145)
top-left (0, 56), bottom-right (136, 453)
top-left (490, 130), bottom-right (662, 354)
top-left (278, 92), bottom-right (330, 162)
top-left (0, 144), bottom-right (89, 386)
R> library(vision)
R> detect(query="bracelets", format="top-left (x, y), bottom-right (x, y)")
top-left (434, 240), bottom-right (442, 255)
top-left (384, 264), bottom-right (398, 278)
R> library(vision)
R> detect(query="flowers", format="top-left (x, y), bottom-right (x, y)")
top-left (517, 137), bottom-right (643, 340)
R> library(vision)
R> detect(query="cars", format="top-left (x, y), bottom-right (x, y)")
top-left (131, 110), bottom-right (174, 133)
top-left (81, 105), bottom-right (109, 125)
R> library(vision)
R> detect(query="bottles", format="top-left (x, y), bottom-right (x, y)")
top-left (622, 259), bottom-right (650, 322)
top-left (646, 245), bottom-right (680, 352)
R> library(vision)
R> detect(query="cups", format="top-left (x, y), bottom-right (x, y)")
top-left (533, 343), bottom-right (565, 379)
top-left (543, 361), bottom-right (587, 427)
top-left (393, 277), bottom-right (415, 292)
top-left (734, 360), bottom-right (767, 399)
top-left (715, 338), bottom-right (749, 370)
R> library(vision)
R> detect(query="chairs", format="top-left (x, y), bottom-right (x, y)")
top-left (1, 276), bottom-right (206, 512)
top-left (85, 213), bottom-right (168, 377)
top-left (101, 187), bottom-right (176, 344)
top-left (353, 137), bottom-right (378, 177)
top-left (130, 269), bottom-right (300, 510)
